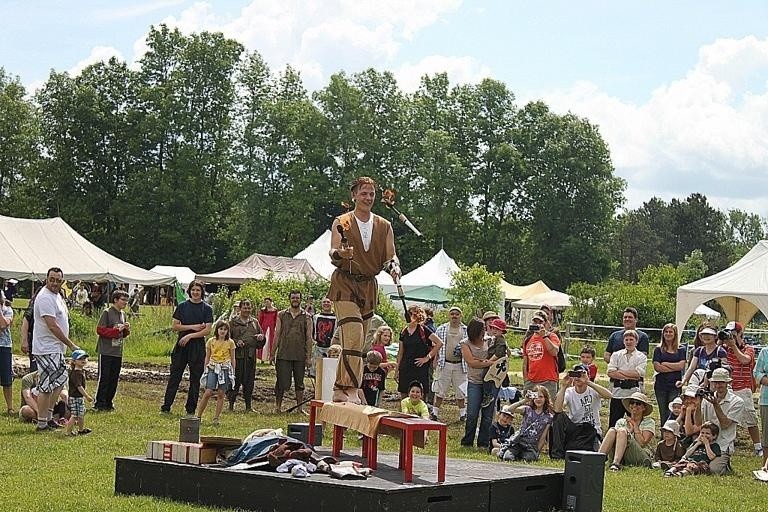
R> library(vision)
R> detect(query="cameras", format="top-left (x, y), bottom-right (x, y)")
top-left (695, 388), bottom-right (713, 398)
top-left (529, 325), bottom-right (540, 331)
top-left (719, 329), bottom-right (734, 341)
top-left (568, 371), bottom-right (581, 377)
top-left (528, 391), bottom-right (539, 399)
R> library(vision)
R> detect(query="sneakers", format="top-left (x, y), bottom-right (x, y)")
top-left (460, 415), bottom-right (468, 421)
top-left (480, 394), bottom-right (494, 407)
top-left (38, 419), bottom-right (91, 435)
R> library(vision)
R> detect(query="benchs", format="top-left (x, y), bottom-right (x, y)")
top-left (306, 400), bottom-right (448, 485)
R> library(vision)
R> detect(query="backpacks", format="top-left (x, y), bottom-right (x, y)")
top-left (402, 324), bottom-right (437, 370)
top-left (525, 330), bottom-right (566, 373)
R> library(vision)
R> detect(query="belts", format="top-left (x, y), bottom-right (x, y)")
top-left (444, 359), bottom-right (461, 364)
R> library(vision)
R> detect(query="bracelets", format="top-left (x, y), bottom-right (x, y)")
top-left (332, 250), bottom-right (342, 261)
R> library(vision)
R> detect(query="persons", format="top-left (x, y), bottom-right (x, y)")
top-left (597, 307), bottom-right (768, 481)
top-left (18, 279), bottom-right (339, 425)
top-left (30, 266), bottom-right (83, 431)
top-left (357, 305), bottom-right (614, 463)
top-left (327, 177), bottom-right (400, 406)
top-left (1, 291), bottom-right (14, 418)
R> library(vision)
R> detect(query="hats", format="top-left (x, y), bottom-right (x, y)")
top-left (501, 405), bottom-right (516, 419)
top-left (408, 380), bottom-right (423, 389)
top-left (449, 306), bottom-right (554, 331)
top-left (72, 350), bottom-right (89, 359)
top-left (622, 319), bottom-right (743, 436)
top-left (574, 363), bottom-right (588, 371)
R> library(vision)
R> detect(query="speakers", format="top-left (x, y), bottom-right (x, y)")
top-left (562, 450), bottom-right (607, 511)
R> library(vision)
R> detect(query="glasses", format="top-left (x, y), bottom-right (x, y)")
top-left (121, 298), bottom-right (129, 302)
top-left (47, 276), bottom-right (64, 283)
